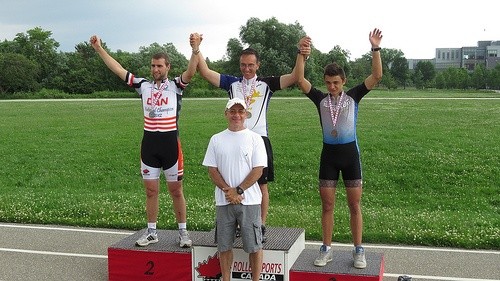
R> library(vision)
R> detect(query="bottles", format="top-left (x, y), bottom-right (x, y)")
top-left (398, 275), bottom-right (412, 281)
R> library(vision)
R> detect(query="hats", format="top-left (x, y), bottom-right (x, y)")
top-left (226, 98), bottom-right (247, 109)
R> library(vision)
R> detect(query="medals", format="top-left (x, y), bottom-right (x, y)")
top-left (331, 130), bottom-right (337, 136)
top-left (149, 111), bottom-right (155, 117)
top-left (245, 112), bottom-right (252, 119)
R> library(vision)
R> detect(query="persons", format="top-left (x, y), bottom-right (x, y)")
top-left (296, 29), bottom-right (382, 267)
top-left (190, 34), bottom-right (311, 243)
top-left (202, 97), bottom-right (267, 281)
top-left (91, 33), bottom-right (204, 248)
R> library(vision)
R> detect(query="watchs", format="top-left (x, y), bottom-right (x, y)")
top-left (236, 186), bottom-right (244, 195)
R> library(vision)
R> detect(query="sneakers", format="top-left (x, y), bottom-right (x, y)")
top-left (179, 229), bottom-right (192, 247)
top-left (353, 246), bottom-right (366, 268)
top-left (136, 228), bottom-right (158, 247)
top-left (313, 245), bottom-right (332, 266)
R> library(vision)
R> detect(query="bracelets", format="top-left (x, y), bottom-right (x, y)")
top-left (192, 50), bottom-right (200, 55)
top-left (297, 50), bottom-right (301, 53)
top-left (305, 56), bottom-right (309, 61)
top-left (371, 48), bottom-right (381, 51)
